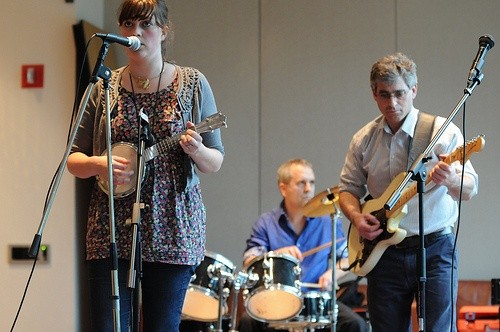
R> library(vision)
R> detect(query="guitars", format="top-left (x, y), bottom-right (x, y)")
top-left (347, 133), bottom-right (485, 278)
top-left (96, 111), bottom-right (229, 200)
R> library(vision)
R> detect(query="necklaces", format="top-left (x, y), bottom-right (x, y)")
top-left (128, 61), bottom-right (165, 90)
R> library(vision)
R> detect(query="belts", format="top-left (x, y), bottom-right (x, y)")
top-left (387, 227), bottom-right (451, 249)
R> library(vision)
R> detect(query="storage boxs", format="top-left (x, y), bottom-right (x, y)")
top-left (458, 305), bottom-right (500, 332)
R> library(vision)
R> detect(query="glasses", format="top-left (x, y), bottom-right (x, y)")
top-left (375, 89), bottom-right (410, 99)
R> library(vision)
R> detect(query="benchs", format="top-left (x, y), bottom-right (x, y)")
top-left (335, 279), bottom-right (500, 332)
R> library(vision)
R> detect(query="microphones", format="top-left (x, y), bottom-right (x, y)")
top-left (94, 33), bottom-right (140, 51)
top-left (139, 108), bottom-right (155, 147)
top-left (468, 34), bottom-right (495, 81)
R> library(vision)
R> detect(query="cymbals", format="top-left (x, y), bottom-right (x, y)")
top-left (302, 186), bottom-right (341, 218)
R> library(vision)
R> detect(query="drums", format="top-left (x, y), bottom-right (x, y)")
top-left (242, 251), bottom-right (304, 323)
top-left (180, 251), bottom-right (237, 322)
top-left (269, 290), bottom-right (338, 329)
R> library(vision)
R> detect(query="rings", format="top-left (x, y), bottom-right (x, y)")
top-left (185, 144), bottom-right (192, 148)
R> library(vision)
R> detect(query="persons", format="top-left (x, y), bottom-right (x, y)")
top-left (242, 159), bottom-right (370, 332)
top-left (65, 0), bottom-right (224, 332)
top-left (338, 52), bottom-right (479, 332)
